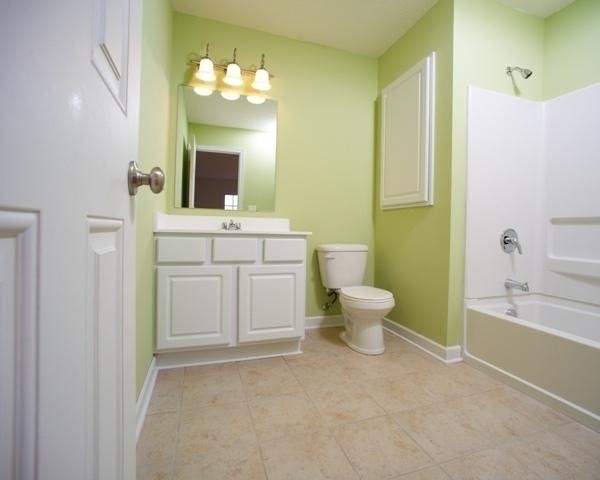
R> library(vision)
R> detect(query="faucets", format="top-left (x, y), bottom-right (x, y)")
top-left (221, 218), bottom-right (242, 231)
top-left (505, 279), bottom-right (531, 293)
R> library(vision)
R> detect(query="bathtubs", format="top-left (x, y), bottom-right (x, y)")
top-left (466, 294), bottom-right (600, 431)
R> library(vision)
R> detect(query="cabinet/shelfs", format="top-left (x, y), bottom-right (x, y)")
top-left (153, 230), bottom-right (311, 367)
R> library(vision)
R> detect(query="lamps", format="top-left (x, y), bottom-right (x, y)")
top-left (191, 44), bottom-right (275, 92)
top-left (193, 85), bottom-right (268, 106)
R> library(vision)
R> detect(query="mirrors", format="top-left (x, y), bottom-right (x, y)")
top-left (174, 85), bottom-right (279, 212)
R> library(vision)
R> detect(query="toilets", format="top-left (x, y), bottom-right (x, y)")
top-left (316, 243), bottom-right (395, 355)
